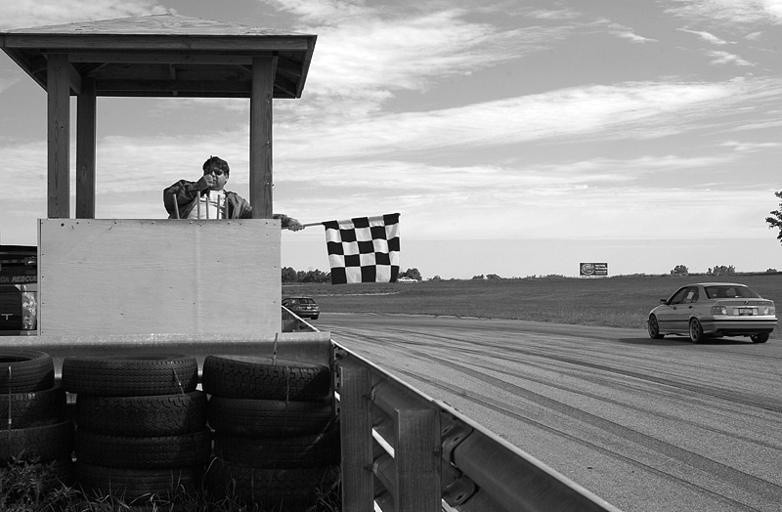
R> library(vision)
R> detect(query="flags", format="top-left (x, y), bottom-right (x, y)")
top-left (324, 211), bottom-right (401, 286)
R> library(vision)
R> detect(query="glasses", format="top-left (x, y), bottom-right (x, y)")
top-left (204, 168), bottom-right (224, 176)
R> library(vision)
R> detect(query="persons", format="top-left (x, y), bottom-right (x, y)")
top-left (162, 156), bottom-right (305, 232)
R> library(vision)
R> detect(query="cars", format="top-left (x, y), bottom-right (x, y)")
top-left (645, 281), bottom-right (779, 345)
top-left (282, 296), bottom-right (321, 320)
